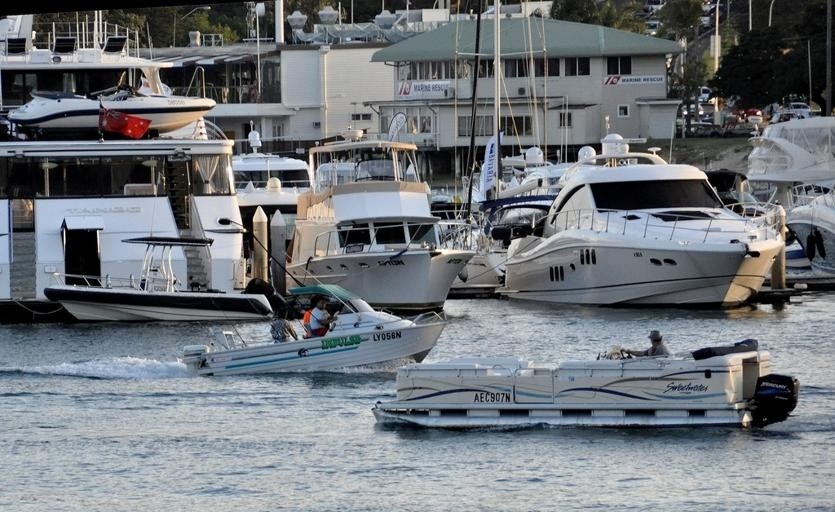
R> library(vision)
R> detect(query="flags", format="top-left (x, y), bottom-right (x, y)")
top-left (99, 107), bottom-right (153, 140)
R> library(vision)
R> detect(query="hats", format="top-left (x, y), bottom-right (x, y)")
top-left (648, 331), bottom-right (663, 340)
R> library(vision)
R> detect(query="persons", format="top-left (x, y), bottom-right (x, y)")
top-left (620, 329), bottom-right (674, 360)
top-left (300, 295), bottom-right (320, 339)
top-left (309, 296), bottom-right (337, 337)
top-left (268, 307), bottom-right (298, 343)
